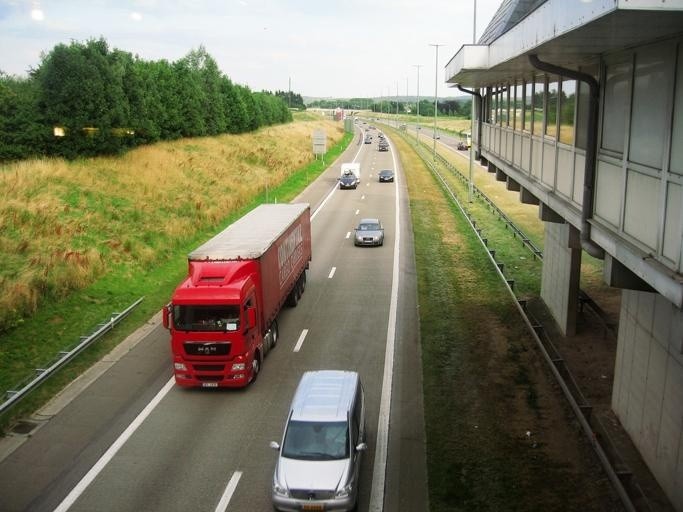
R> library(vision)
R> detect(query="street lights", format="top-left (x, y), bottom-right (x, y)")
top-left (428, 43), bottom-right (445, 157)
top-left (414, 64), bottom-right (424, 143)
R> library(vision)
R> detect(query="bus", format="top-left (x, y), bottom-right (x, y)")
top-left (461, 132), bottom-right (471, 147)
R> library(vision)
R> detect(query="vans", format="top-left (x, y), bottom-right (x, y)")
top-left (269, 369), bottom-right (367, 512)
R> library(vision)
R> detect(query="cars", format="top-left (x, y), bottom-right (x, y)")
top-left (378, 169), bottom-right (394, 182)
top-left (457, 142), bottom-right (468, 151)
top-left (354, 218), bottom-right (384, 246)
top-left (337, 172), bottom-right (358, 190)
top-left (364, 132), bottom-right (389, 151)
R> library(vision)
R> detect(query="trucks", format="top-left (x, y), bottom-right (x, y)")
top-left (162, 203), bottom-right (312, 390)
